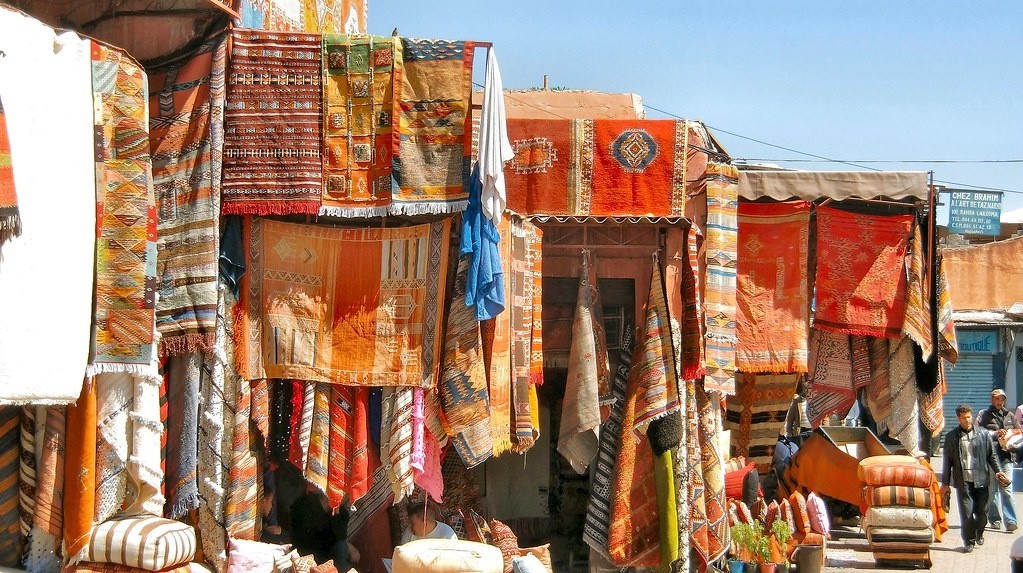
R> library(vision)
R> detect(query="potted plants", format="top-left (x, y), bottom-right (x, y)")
top-left (728, 522), bottom-right (744, 573)
top-left (770, 519), bottom-right (791, 573)
top-left (743, 519), bottom-right (765, 573)
top-left (759, 535), bottom-right (776, 573)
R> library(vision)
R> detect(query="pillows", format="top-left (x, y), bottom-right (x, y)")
top-left (227, 537), bottom-right (292, 573)
top-left (390, 540), bottom-right (505, 573)
top-left (779, 497), bottom-right (796, 534)
top-left (81, 516), bottom-right (197, 571)
top-left (865, 486), bottom-right (934, 508)
top-left (272, 547), bottom-right (300, 573)
top-left (767, 499), bottom-right (782, 526)
top-left (752, 498), bottom-right (769, 524)
top-left (460, 509), bottom-right (493, 545)
top-left (864, 466), bottom-right (934, 488)
top-left (519, 542), bottom-right (552, 573)
top-left (807, 492), bottom-right (829, 534)
top-left (490, 518), bottom-right (520, 572)
top-left (727, 497), bottom-right (739, 526)
top-left (511, 552), bottom-right (547, 573)
top-left (865, 505), bottom-right (933, 528)
top-left (857, 455), bottom-right (923, 482)
top-left (736, 500), bottom-right (753, 528)
top-left (310, 560), bottom-right (338, 573)
top-left (289, 553), bottom-right (318, 573)
top-left (859, 481), bottom-right (869, 501)
top-left (788, 491), bottom-right (811, 536)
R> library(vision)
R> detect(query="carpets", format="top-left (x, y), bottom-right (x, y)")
top-left (0, 0), bottom-right (948, 573)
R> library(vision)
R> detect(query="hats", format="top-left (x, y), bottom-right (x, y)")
top-left (992, 389), bottom-right (1008, 399)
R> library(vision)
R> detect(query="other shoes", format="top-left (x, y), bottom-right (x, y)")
top-left (991, 521), bottom-right (1000, 529)
top-left (975, 532), bottom-right (984, 546)
top-left (964, 542), bottom-right (974, 552)
top-left (1006, 522), bottom-right (1018, 531)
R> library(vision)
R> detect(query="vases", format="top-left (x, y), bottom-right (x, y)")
top-left (793, 544), bottom-right (823, 573)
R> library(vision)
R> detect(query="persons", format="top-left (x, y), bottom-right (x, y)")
top-left (262, 481), bottom-right (290, 545)
top-left (401, 502), bottom-right (458, 545)
top-left (1010, 536), bottom-right (1023, 573)
top-left (287, 474), bottom-right (360, 573)
top-left (772, 435), bottom-right (800, 477)
top-left (940, 403), bottom-right (1007, 552)
top-left (974, 389), bottom-right (1023, 534)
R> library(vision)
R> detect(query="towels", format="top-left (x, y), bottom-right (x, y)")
top-left (460, 160), bottom-right (504, 320)
top-left (479, 47), bottom-right (515, 227)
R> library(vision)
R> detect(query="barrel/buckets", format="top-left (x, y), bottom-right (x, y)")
top-left (795, 544), bottom-right (823, 573)
